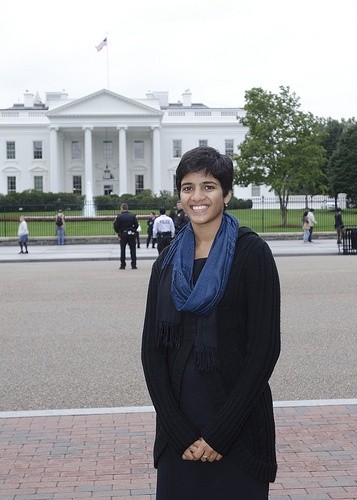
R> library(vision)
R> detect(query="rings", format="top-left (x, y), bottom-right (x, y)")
top-left (202, 456), bottom-right (209, 460)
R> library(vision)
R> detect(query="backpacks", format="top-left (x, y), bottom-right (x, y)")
top-left (56, 215), bottom-right (63, 226)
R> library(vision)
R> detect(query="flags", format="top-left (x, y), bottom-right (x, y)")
top-left (96, 37), bottom-right (107, 51)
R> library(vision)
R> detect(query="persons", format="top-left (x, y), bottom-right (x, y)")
top-left (335, 208), bottom-right (345, 244)
top-left (111, 197), bottom-right (192, 270)
top-left (56, 209), bottom-right (65, 245)
top-left (301, 209), bottom-right (317, 243)
top-left (17, 215), bottom-right (28, 254)
top-left (141, 147), bottom-right (280, 500)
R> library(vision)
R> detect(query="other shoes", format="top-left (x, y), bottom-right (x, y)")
top-left (19, 251), bottom-right (23, 254)
top-left (120, 266), bottom-right (125, 269)
top-left (132, 266), bottom-right (137, 269)
top-left (23, 251), bottom-right (28, 254)
